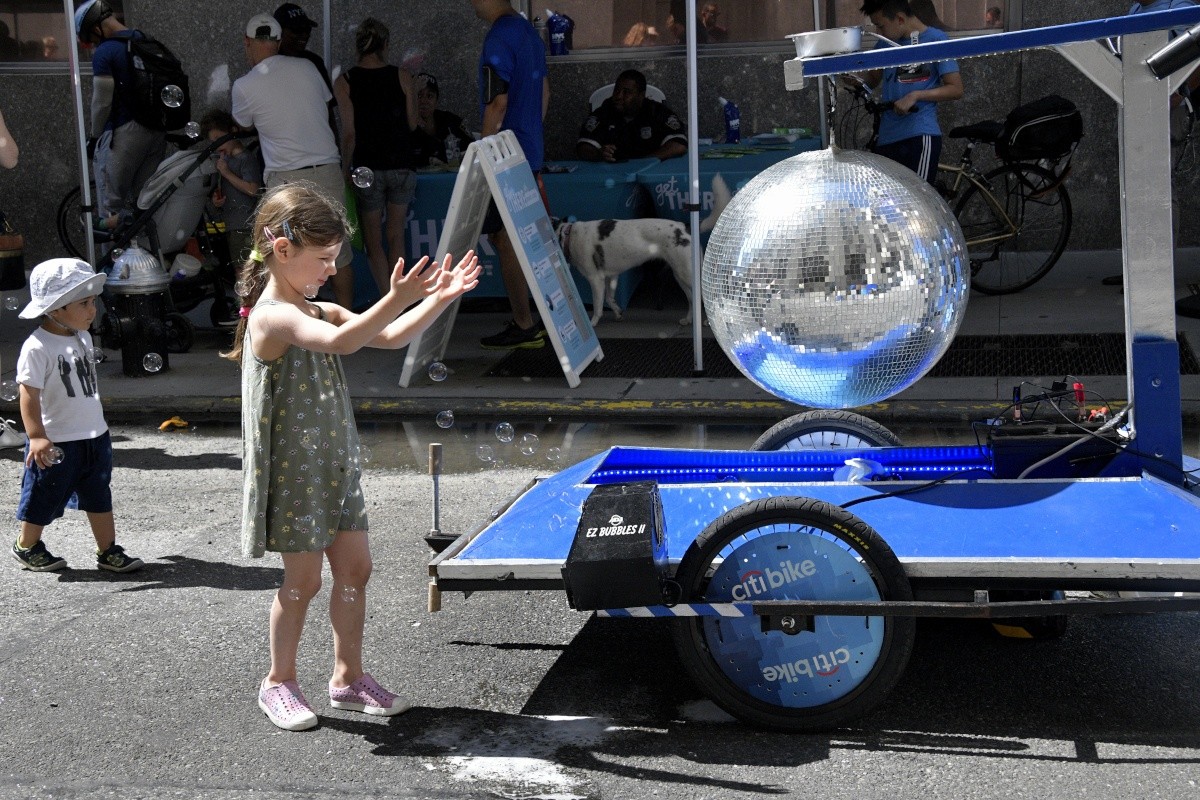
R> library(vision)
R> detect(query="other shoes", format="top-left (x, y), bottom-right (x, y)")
top-left (216, 304), bottom-right (242, 326)
top-left (79, 214), bottom-right (109, 232)
top-left (1175, 294), bottom-right (1200, 319)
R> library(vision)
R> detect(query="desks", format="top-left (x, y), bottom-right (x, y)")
top-left (349, 130), bottom-right (830, 311)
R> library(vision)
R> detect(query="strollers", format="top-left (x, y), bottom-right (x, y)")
top-left (83, 130), bottom-right (263, 358)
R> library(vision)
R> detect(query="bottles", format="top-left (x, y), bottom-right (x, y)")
top-left (169, 253), bottom-right (202, 282)
top-left (533, 17), bottom-right (550, 56)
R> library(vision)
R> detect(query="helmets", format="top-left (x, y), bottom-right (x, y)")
top-left (74, 0), bottom-right (113, 45)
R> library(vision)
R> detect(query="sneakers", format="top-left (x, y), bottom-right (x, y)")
top-left (10, 533), bottom-right (67, 572)
top-left (0, 417), bottom-right (27, 450)
top-left (97, 541), bottom-right (145, 573)
top-left (479, 317), bottom-right (548, 349)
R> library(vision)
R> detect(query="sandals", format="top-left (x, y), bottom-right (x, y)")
top-left (258, 678), bottom-right (319, 731)
top-left (329, 671), bottom-right (410, 715)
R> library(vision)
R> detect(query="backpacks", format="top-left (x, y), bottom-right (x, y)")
top-left (97, 28), bottom-right (191, 131)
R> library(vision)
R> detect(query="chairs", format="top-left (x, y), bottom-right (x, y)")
top-left (1003, 95), bottom-right (1083, 165)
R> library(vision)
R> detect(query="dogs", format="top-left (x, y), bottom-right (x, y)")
top-left (545, 217), bottom-right (711, 325)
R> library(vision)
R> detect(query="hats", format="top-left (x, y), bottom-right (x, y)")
top-left (274, 3), bottom-right (319, 28)
top-left (17, 257), bottom-right (108, 320)
top-left (647, 26), bottom-right (659, 42)
top-left (245, 13), bottom-right (282, 41)
top-left (412, 73), bottom-right (438, 92)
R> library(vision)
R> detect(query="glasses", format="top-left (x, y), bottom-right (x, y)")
top-left (986, 20), bottom-right (991, 24)
top-left (704, 11), bottom-right (720, 17)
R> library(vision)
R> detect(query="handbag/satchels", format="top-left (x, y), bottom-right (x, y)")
top-left (994, 94), bottom-right (1085, 199)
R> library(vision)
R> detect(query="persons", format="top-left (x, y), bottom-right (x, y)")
top-left (660, 0), bottom-right (729, 47)
top-left (200, 109), bottom-right (261, 325)
top-left (842, 0), bottom-right (965, 186)
top-left (11, 257), bottom-right (144, 572)
top-left (0, 110), bottom-right (20, 170)
top-left (984, 7), bottom-right (1003, 27)
top-left (908, 0), bottom-right (959, 31)
top-left (275, 2), bottom-right (343, 167)
top-left (410, 72), bottom-right (477, 168)
top-left (1102, 0), bottom-right (1200, 295)
top-left (333, 18), bottom-right (419, 297)
top-left (573, 69), bottom-right (689, 162)
top-left (75, 0), bottom-right (167, 259)
top-left (218, 180), bottom-right (482, 732)
top-left (231, 15), bottom-right (355, 315)
top-left (471, 0), bottom-right (549, 351)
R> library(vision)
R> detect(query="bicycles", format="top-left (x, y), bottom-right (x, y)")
top-left (835, 101), bottom-right (1075, 296)
top-left (54, 132), bottom-right (208, 269)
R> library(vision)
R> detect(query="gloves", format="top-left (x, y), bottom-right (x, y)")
top-left (86, 136), bottom-right (99, 160)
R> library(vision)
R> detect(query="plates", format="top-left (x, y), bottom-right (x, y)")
top-left (445, 165), bottom-right (460, 172)
top-left (415, 167), bottom-right (442, 174)
